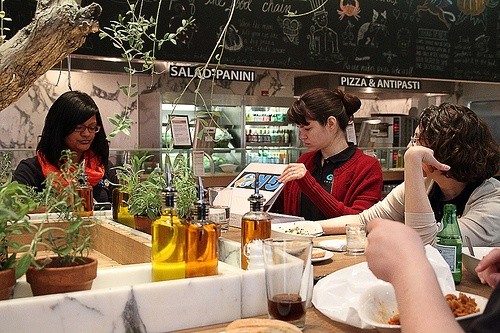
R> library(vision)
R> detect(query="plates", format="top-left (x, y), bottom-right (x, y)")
top-left (311, 248), bottom-right (334, 263)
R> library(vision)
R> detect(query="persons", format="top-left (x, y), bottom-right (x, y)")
top-left (322, 103), bottom-right (500, 333)
top-left (11, 90), bottom-right (119, 211)
top-left (269, 88), bottom-right (383, 221)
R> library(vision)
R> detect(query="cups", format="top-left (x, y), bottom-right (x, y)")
top-left (262, 237), bottom-right (314, 330)
top-left (344, 223), bottom-right (367, 256)
top-left (208, 205), bottom-right (230, 234)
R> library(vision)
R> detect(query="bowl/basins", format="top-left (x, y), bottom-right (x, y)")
top-left (219, 163), bottom-right (239, 173)
top-left (462, 246), bottom-right (496, 274)
top-left (358, 290), bottom-right (489, 333)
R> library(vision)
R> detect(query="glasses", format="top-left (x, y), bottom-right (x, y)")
top-left (411, 136), bottom-right (419, 142)
top-left (74, 123), bottom-right (101, 133)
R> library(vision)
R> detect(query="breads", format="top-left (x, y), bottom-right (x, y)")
top-left (278, 241), bottom-right (326, 258)
top-left (225, 318), bottom-right (303, 333)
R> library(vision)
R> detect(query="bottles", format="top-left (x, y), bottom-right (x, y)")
top-left (246, 128), bottom-right (293, 142)
top-left (186, 177), bottom-right (220, 278)
top-left (246, 113), bottom-right (288, 121)
top-left (111, 153), bottom-right (135, 231)
top-left (396, 150), bottom-right (404, 168)
top-left (150, 165), bottom-right (186, 282)
top-left (436, 204), bottom-right (462, 284)
top-left (240, 173), bottom-right (271, 271)
top-left (381, 185), bottom-right (399, 200)
top-left (246, 152), bottom-right (288, 165)
top-left (71, 160), bottom-right (93, 218)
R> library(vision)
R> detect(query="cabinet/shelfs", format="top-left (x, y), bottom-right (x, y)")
top-left (138, 92), bottom-right (243, 187)
top-left (244, 95), bottom-right (299, 170)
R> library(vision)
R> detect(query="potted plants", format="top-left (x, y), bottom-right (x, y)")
top-left (0, 148), bottom-right (199, 301)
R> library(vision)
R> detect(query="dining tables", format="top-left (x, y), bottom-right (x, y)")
top-left (172, 226), bottom-right (500, 333)
